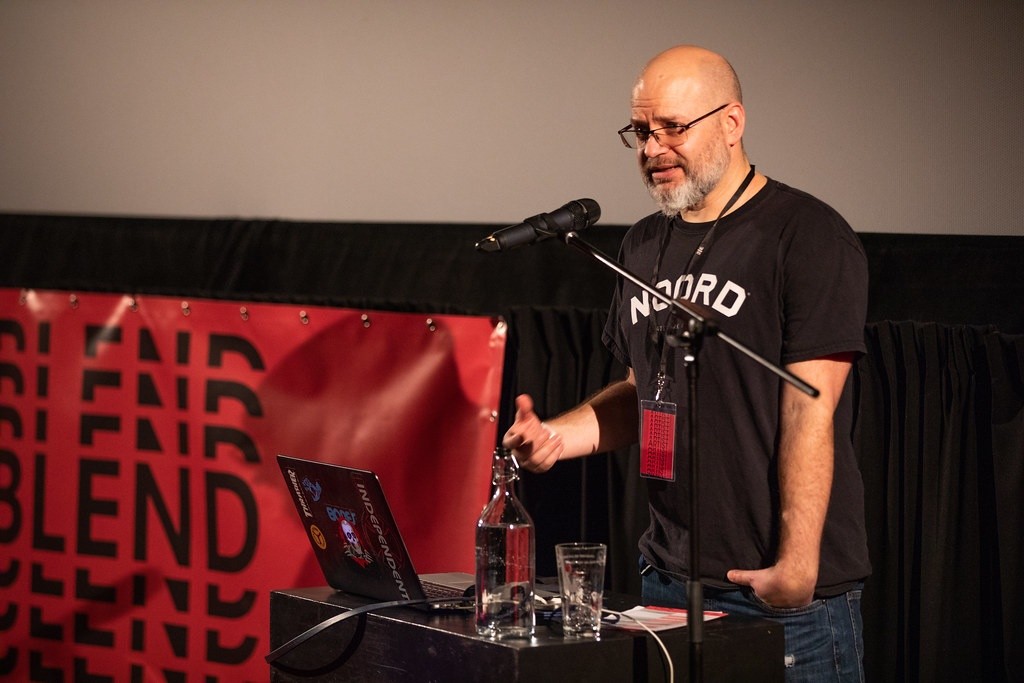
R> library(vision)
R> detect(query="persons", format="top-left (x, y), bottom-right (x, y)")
top-left (501, 43), bottom-right (869, 683)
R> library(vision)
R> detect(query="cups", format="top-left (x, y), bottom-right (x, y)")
top-left (558, 544), bottom-right (606, 642)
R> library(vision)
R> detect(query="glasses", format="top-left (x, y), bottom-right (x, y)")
top-left (617, 102), bottom-right (732, 149)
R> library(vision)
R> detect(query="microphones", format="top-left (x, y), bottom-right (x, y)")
top-left (476, 199), bottom-right (602, 254)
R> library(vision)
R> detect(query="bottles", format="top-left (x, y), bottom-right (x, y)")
top-left (474, 448), bottom-right (535, 639)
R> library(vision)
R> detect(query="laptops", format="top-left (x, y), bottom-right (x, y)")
top-left (277, 455), bottom-right (558, 612)
